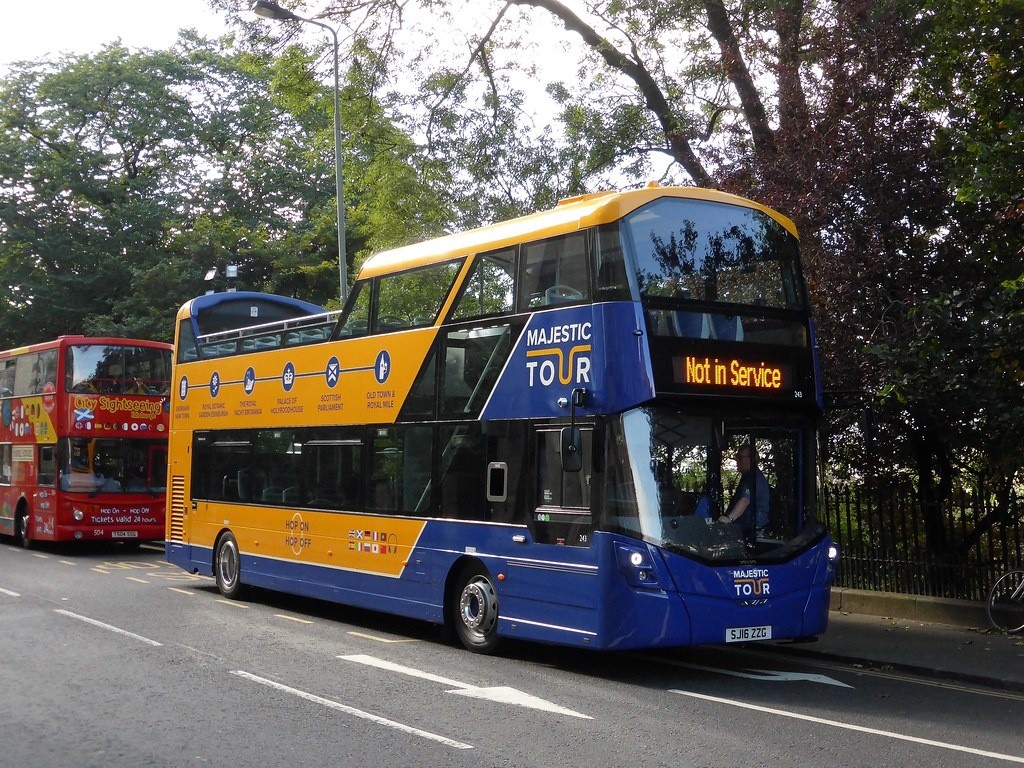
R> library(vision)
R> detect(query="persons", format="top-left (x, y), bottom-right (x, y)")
top-left (658, 472), bottom-right (680, 515)
top-left (715, 446), bottom-right (770, 538)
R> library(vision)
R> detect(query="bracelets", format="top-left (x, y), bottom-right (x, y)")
top-left (726, 516), bottom-right (734, 523)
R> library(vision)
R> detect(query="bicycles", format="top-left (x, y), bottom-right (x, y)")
top-left (986, 566), bottom-right (1024, 635)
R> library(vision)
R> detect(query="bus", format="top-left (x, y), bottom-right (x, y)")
top-left (163, 183), bottom-right (869, 659)
top-left (0, 335), bottom-right (167, 549)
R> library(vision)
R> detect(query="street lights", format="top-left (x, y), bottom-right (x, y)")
top-left (252, 0), bottom-right (349, 317)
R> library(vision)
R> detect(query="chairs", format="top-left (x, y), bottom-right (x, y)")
top-left (222, 469), bottom-right (296, 502)
top-left (544, 285), bottom-right (584, 305)
top-left (184, 316), bottom-right (434, 362)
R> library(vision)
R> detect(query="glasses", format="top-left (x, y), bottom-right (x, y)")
top-left (734, 455), bottom-right (750, 462)
